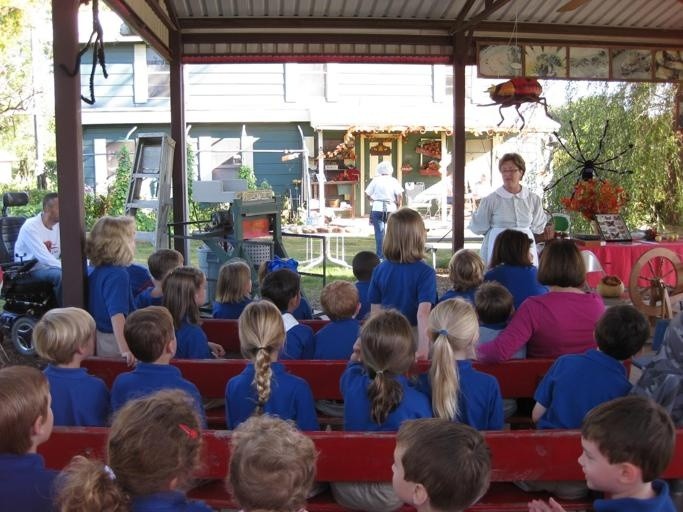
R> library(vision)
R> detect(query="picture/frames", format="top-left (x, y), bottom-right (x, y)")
top-left (593, 212), bottom-right (632, 242)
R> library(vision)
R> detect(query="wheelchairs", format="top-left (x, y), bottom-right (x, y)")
top-left (0, 192), bottom-right (52, 358)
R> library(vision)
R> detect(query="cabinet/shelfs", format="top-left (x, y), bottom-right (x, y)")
top-left (309, 155), bottom-right (357, 219)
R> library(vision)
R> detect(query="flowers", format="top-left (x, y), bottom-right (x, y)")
top-left (560, 175), bottom-right (626, 221)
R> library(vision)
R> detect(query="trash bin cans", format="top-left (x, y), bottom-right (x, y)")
top-left (197, 245), bottom-right (223, 304)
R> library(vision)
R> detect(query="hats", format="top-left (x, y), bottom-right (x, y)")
top-left (377, 161), bottom-right (393, 174)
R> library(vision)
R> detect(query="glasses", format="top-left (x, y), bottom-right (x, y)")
top-left (501, 168), bottom-right (519, 173)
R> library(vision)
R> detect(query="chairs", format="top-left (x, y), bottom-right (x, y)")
top-left (0, 192), bottom-right (54, 296)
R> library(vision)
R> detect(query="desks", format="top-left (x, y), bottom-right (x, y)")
top-left (573, 238), bottom-right (683, 290)
top-left (278, 199), bottom-right (485, 274)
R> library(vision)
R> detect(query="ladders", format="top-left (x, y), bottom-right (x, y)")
top-left (124, 132), bottom-right (176, 281)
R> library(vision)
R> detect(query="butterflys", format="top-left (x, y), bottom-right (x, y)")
top-left (598, 216), bottom-right (629, 241)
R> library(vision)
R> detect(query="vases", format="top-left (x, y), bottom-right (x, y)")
top-left (590, 219), bottom-right (601, 238)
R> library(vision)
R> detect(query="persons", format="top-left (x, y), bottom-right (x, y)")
top-left (366, 161), bottom-right (405, 261)
top-left (1, 193), bottom-right (682, 512)
top-left (471, 154), bottom-right (547, 268)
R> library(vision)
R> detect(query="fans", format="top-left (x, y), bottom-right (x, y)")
top-left (557, 0), bottom-right (589, 12)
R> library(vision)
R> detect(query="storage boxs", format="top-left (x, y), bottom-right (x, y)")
top-left (197, 245), bottom-right (233, 280)
